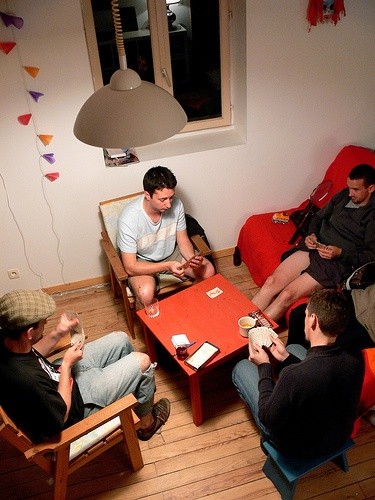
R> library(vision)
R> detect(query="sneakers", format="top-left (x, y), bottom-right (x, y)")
top-left (138, 397), bottom-right (170, 441)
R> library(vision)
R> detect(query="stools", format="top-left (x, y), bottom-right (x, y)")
top-left (261, 439), bottom-right (355, 500)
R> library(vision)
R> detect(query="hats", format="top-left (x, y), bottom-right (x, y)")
top-left (0, 289), bottom-right (57, 328)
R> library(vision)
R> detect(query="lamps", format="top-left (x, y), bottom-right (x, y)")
top-left (72, 0), bottom-right (188, 149)
top-left (165, 0), bottom-right (181, 32)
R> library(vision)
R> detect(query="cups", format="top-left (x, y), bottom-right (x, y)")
top-left (144, 297), bottom-right (159, 318)
top-left (239, 316), bottom-right (259, 338)
top-left (175, 341), bottom-right (188, 358)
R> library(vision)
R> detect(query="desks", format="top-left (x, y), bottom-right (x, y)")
top-left (113, 23), bottom-right (187, 57)
top-left (135, 273), bottom-right (279, 427)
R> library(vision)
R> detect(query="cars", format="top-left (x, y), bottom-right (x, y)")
top-left (272, 212), bottom-right (289, 225)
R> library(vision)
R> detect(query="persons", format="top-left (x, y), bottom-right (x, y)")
top-left (232, 284), bottom-right (375, 456)
top-left (117, 165), bottom-right (215, 312)
top-left (251, 163), bottom-right (375, 322)
top-left (0, 289), bottom-right (170, 455)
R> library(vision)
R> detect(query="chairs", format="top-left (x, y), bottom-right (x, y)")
top-left (0, 333), bottom-right (144, 500)
top-left (98, 191), bottom-right (212, 339)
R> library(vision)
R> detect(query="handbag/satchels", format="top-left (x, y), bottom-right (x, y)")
top-left (292, 201), bottom-right (321, 236)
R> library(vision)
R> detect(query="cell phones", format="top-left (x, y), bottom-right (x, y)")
top-left (184, 341), bottom-right (220, 373)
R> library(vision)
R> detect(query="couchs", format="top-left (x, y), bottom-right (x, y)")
top-left (233, 145), bottom-right (375, 438)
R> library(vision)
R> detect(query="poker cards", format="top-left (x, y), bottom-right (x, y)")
top-left (178, 250), bottom-right (203, 269)
top-left (64, 310), bottom-right (86, 349)
top-left (248, 325), bottom-right (279, 350)
top-left (205, 287), bottom-right (223, 299)
top-left (308, 235), bottom-right (331, 250)
top-left (171, 334), bottom-right (190, 349)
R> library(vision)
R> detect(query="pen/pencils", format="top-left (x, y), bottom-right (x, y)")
top-left (172, 340), bottom-right (196, 356)
